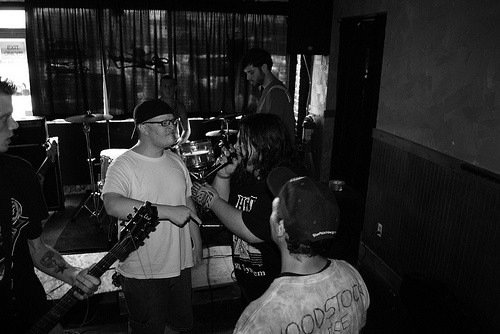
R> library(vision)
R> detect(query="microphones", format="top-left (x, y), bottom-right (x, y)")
top-left (202, 152), bottom-right (238, 181)
top-left (152, 57), bottom-right (167, 74)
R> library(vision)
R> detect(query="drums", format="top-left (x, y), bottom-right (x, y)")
top-left (179, 139), bottom-right (216, 163)
top-left (183, 151), bottom-right (209, 170)
top-left (99, 149), bottom-right (130, 194)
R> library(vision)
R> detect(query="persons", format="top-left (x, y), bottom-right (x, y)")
top-left (0, 80), bottom-right (101, 334)
top-left (240, 48), bottom-right (297, 148)
top-left (100, 98), bottom-right (203, 334)
top-left (232, 173), bottom-right (371, 334)
top-left (158, 75), bottom-right (191, 145)
top-left (191, 114), bottom-right (308, 304)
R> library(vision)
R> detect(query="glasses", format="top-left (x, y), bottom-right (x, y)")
top-left (142, 117), bottom-right (181, 128)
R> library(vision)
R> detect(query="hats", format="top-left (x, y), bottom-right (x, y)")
top-left (130, 99), bottom-right (173, 140)
top-left (267, 167), bottom-right (339, 243)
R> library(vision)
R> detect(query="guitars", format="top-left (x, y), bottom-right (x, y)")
top-left (25, 201), bottom-right (160, 334)
top-left (36, 140), bottom-right (58, 183)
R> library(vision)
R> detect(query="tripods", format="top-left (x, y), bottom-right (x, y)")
top-left (70, 124), bottom-right (105, 232)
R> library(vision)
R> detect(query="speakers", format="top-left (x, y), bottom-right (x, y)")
top-left (9, 120), bottom-right (66, 213)
top-left (287, 0), bottom-right (335, 56)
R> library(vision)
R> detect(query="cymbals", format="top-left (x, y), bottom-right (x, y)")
top-left (205, 128), bottom-right (237, 136)
top-left (204, 112), bottom-right (243, 123)
top-left (64, 114), bottom-right (113, 124)
